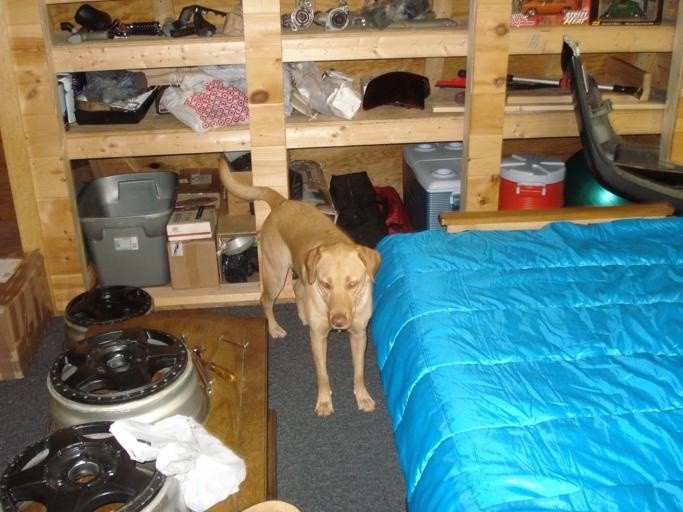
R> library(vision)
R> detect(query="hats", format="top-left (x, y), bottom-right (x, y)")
top-left (362, 71), bottom-right (430, 110)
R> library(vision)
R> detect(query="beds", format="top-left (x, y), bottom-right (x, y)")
top-left (363, 209), bottom-right (682, 512)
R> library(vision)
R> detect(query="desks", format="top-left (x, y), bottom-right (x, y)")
top-left (23, 306), bottom-right (289, 512)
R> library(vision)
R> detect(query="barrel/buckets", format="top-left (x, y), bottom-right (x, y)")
top-left (499, 150), bottom-right (568, 212)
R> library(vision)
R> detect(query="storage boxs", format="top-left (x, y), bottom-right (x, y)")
top-left (0, 244), bottom-right (50, 387)
top-left (74, 167), bottom-right (220, 291)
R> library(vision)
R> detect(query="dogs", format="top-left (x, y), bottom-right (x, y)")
top-left (217, 156), bottom-right (381, 417)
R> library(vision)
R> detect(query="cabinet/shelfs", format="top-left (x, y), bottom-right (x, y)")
top-left (0, 2), bottom-right (683, 315)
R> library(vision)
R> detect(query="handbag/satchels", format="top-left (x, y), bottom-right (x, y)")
top-left (329, 170), bottom-right (401, 249)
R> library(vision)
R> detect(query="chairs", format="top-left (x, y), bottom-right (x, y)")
top-left (552, 33), bottom-right (682, 218)
